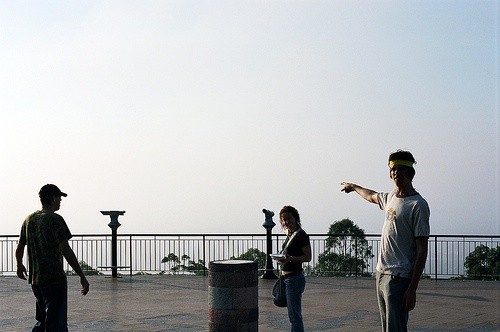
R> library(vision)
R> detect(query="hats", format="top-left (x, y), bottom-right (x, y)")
top-left (38, 184), bottom-right (68, 197)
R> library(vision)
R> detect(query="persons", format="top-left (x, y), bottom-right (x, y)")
top-left (340, 150), bottom-right (431, 332)
top-left (269, 206), bottom-right (312, 332)
top-left (15, 184), bottom-right (89, 332)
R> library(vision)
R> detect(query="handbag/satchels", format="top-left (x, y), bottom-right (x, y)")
top-left (271, 277), bottom-right (288, 307)
